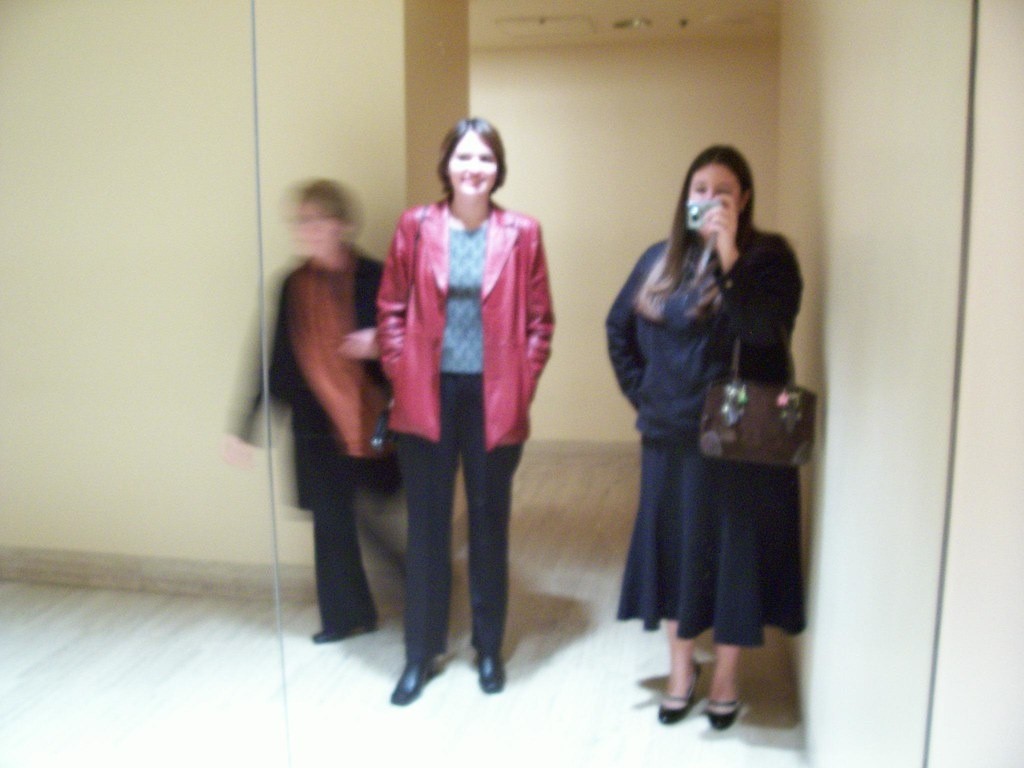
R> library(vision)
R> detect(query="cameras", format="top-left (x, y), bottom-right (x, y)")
top-left (686, 199), bottom-right (722, 230)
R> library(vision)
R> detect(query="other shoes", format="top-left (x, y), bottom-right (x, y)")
top-left (314, 618), bottom-right (376, 643)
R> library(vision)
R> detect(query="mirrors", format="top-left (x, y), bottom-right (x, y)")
top-left (253, 0), bottom-right (979, 768)
top-left (0, 0), bottom-right (291, 768)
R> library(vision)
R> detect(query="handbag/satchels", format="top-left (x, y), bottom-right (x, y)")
top-left (370, 409), bottom-right (399, 474)
top-left (698, 324), bottom-right (816, 467)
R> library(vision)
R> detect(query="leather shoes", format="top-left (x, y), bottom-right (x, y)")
top-left (392, 652), bottom-right (445, 704)
top-left (478, 652), bottom-right (501, 692)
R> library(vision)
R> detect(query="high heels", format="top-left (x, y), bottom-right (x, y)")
top-left (706, 700), bottom-right (737, 729)
top-left (659, 663), bottom-right (700, 724)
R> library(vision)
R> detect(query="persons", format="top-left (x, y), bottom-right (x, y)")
top-left (267, 181), bottom-right (407, 642)
top-left (377, 118), bottom-right (554, 705)
top-left (607, 145), bottom-right (807, 730)
